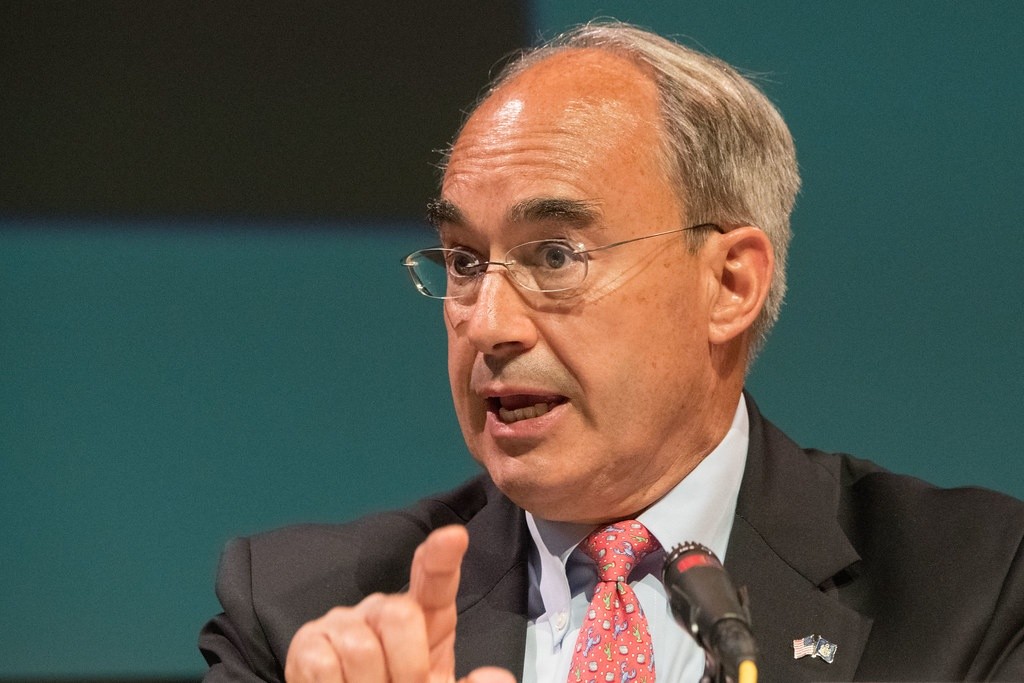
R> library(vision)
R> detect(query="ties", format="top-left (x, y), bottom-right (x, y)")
top-left (567, 520), bottom-right (660, 683)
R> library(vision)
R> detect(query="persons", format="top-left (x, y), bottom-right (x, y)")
top-left (196, 17), bottom-right (1024, 683)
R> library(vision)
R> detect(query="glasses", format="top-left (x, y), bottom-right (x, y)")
top-left (399, 223), bottom-right (727, 300)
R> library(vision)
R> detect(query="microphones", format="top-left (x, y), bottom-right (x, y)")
top-left (661, 541), bottom-right (758, 676)
